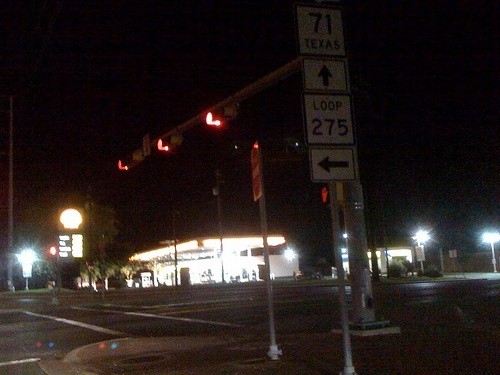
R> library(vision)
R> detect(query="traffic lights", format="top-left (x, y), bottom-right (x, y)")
top-left (115, 157), bottom-right (134, 169)
top-left (155, 138), bottom-right (174, 151)
top-left (204, 113), bottom-right (226, 129)
